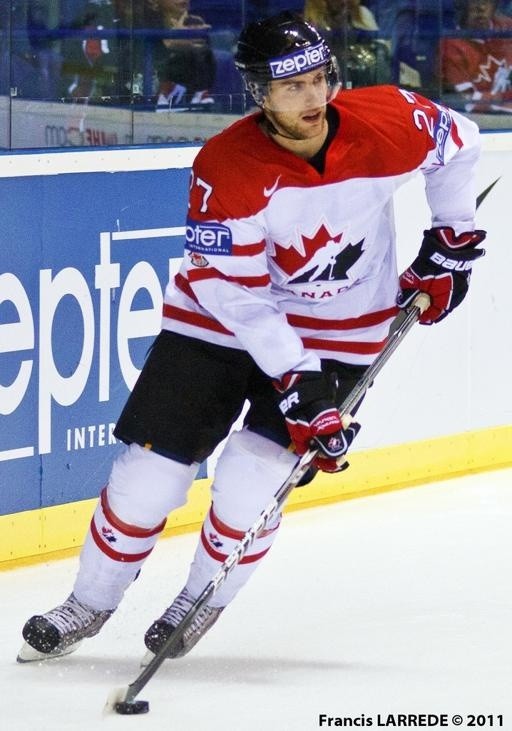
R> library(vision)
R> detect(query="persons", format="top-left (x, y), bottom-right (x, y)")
top-left (46, 1), bottom-right (219, 148)
top-left (432, 1), bottom-right (511, 116)
top-left (304, 1), bottom-right (421, 88)
top-left (116, 0), bottom-right (219, 105)
top-left (19, 14), bottom-right (489, 664)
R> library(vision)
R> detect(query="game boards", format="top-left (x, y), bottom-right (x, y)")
top-left (103, 290), bottom-right (431, 715)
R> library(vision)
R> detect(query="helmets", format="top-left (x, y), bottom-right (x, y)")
top-left (234, 9), bottom-right (343, 106)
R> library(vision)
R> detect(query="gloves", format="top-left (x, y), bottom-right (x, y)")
top-left (394, 226), bottom-right (487, 325)
top-left (271, 370), bottom-right (362, 474)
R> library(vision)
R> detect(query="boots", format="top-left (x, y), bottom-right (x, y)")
top-left (22, 592), bottom-right (118, 655)
top-left (144, 585), bottom-right (227, 659)
